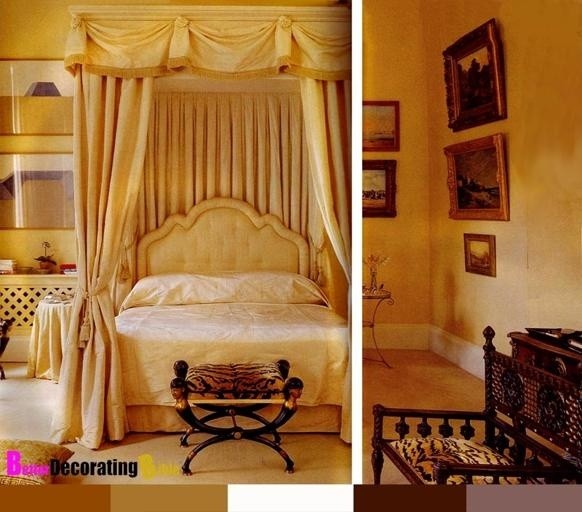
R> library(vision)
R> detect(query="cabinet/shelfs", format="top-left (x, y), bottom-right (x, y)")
top-left (506, 327), bottom-right (582, 390)
top-left (0, 268), bottom-right (78, 365)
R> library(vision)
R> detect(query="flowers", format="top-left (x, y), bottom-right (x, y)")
top-left (33, 240), bottom-right (60, 265)
top-left (361, 252), bottom-right (392, 273)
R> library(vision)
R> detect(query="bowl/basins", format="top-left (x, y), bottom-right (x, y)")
top-left (15, 266), bottom-right (33, 274)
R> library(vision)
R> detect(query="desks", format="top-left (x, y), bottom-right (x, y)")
top-left (25, 294), bottom-right (76, 382)
top-left (361, 286), bottom-right (395, 369)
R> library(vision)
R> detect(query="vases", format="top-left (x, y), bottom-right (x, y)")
top-left (39, 262), bottom-right (51, 269)
top-left (369, 270), bottom-right (379, 293)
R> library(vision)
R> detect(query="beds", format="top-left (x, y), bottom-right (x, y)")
top-left (107, 195), bottom-right (351, 440)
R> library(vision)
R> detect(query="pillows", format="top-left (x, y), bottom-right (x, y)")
top-left (1, 436), bottom-right (78, 487)
top-left (119, 267), bottom-right (327, 312)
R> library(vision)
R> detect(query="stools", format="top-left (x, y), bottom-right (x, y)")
top-left (167, 355), bottom-right (307, 479)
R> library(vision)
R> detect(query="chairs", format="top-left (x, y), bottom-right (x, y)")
top-left (369, 321), bottom-right (582, 485)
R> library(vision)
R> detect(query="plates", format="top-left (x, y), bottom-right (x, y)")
top-left (36, 269), bottom-right (52, 274)
top-left (63, 269), bottom-right (77, 275)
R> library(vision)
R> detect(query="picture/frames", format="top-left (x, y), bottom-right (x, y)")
top-left (462, 231), bottom-right (497, 279)
top-left (0, 56), bottom-right (76, 138)
top-left (440, 15), bottom-right (511, 134)
top-left (444, 132), bottom-right (512, 224)
top-left (361, 157), bottom-right (398, 221)
top-left (361, 99), bottom-right (403, 153)
top-left (0, 148), bottom-right (78, 232)
